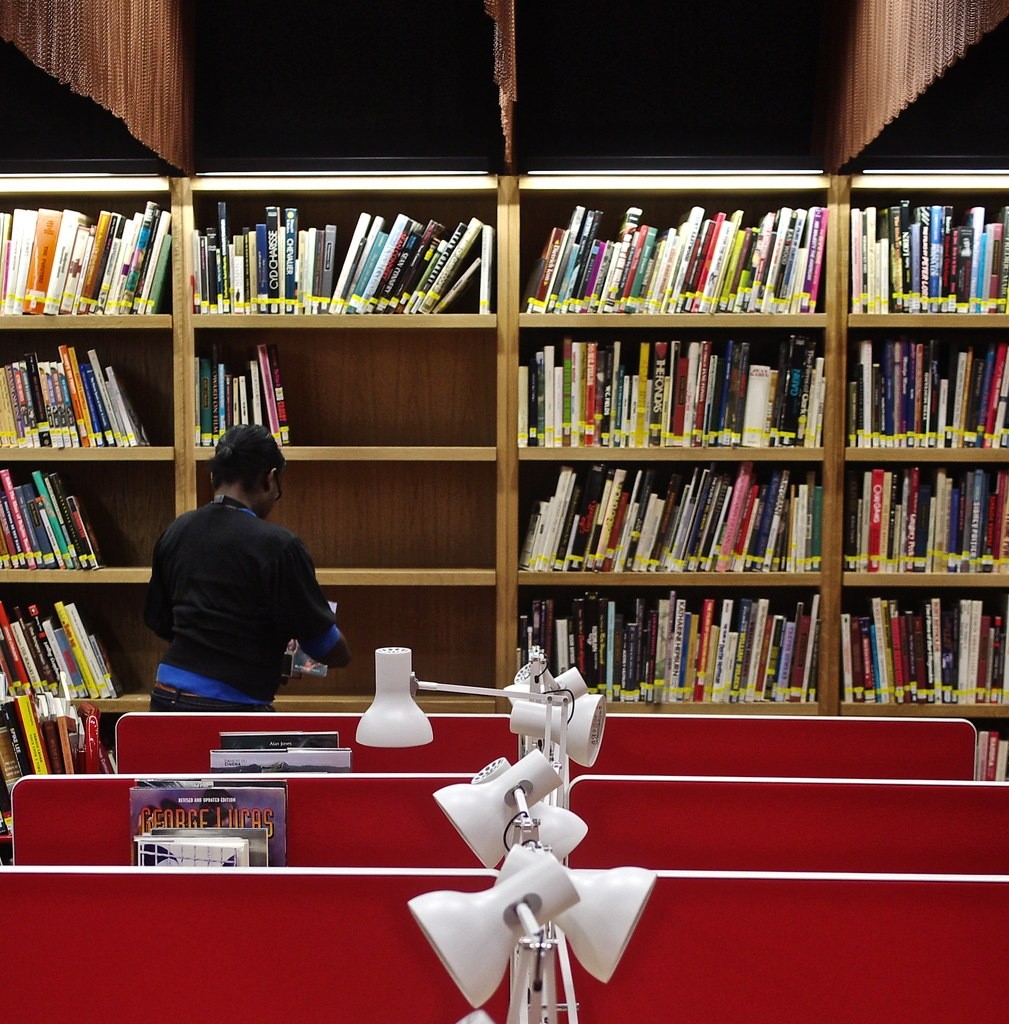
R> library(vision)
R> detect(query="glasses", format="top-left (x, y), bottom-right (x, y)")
top-left (267, 466), bottom-right (283, 501)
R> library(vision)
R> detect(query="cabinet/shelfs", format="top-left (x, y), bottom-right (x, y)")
top-left (0, 170), bottom-right (1009, 720)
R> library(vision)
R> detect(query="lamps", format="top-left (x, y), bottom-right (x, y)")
top-left (354, 648), bottom-right (659, 1022)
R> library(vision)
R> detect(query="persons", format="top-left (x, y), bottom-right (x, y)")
top-left (143, 424), bottom-right (350, 713)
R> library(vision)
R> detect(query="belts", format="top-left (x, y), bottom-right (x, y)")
top-left (155, 682), bottom-right (202, 697)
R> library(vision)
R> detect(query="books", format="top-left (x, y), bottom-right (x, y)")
top-left (195, 342), bottom-right (292, 447)
top-left (0, 201), bottom-right (173, 317)
top-left (128, 779), bottom-right (289, 867)
top-left (210, 731), bottom-right (353, 773)
top-left (518, 463), bottom-right (824, 574)
top-left (516, 334), bottom-right (826, 449)
top-left (193, 201), bottom-right (496, 317)
top-left (0, 467), bottom-right (109, 571)
top-left (840, 595), bottom-right (1009, 704)
top-left (843, 465), bottom-right (1009, 574)
top-left (0, 345), bottom-right (150, 449)
top-left (850, 198), bottom-right (1009, 315)
top-left (0, 691), bottom-right (116, 865)
top-left (977, 730), bottom-right (1009, 780)
top-left (846, 338), bottom-right (1009, 449)
top-left (284, 599), bottom-right (337, 677)
top-left (520, 205), bottom-right (828, 314)
top-left (0, 600), bottom-right (124, 700)
top-left (515, 590), bottom-right (822, 705)
